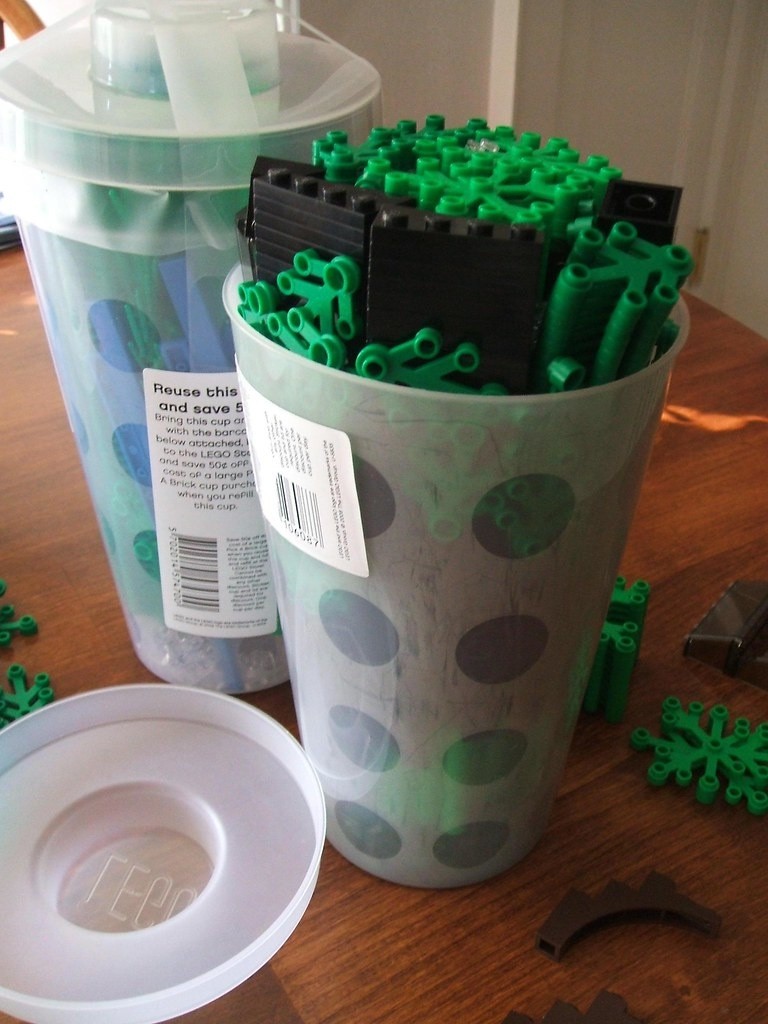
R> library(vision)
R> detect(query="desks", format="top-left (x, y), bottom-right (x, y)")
top-left (0, 239), bottom-right (768, 1023)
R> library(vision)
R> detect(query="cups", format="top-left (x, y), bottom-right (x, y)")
top-left (220, 263), bottom-right (690, 891)
top-left (0, 0), bottom-right (381, 696)
